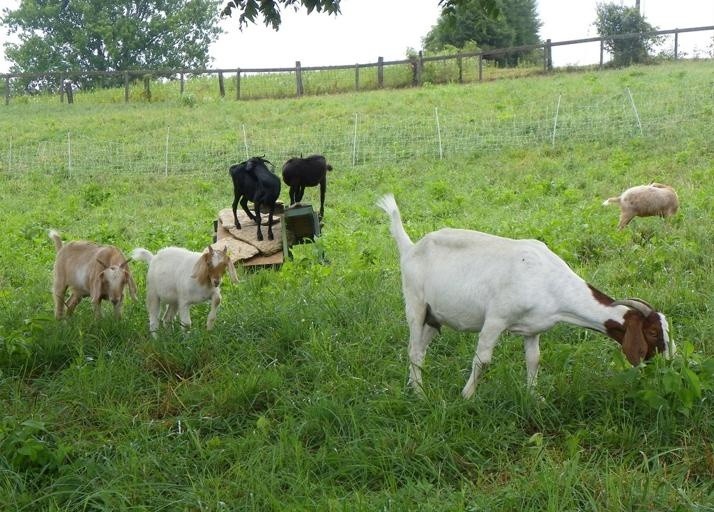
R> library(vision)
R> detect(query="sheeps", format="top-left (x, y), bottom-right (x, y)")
top-left (228, 154), bottom-right (281, 241)
top-left (376, 191), bottom-right (678, 407)
top-left (48, 230), bottom-right (139, 322)
top-left (131, 244), bottom-right (241, 341)
top-left (602, 178), bottom-right (681, 233)
top-left (282, 152), bottom-right (333, 217)
